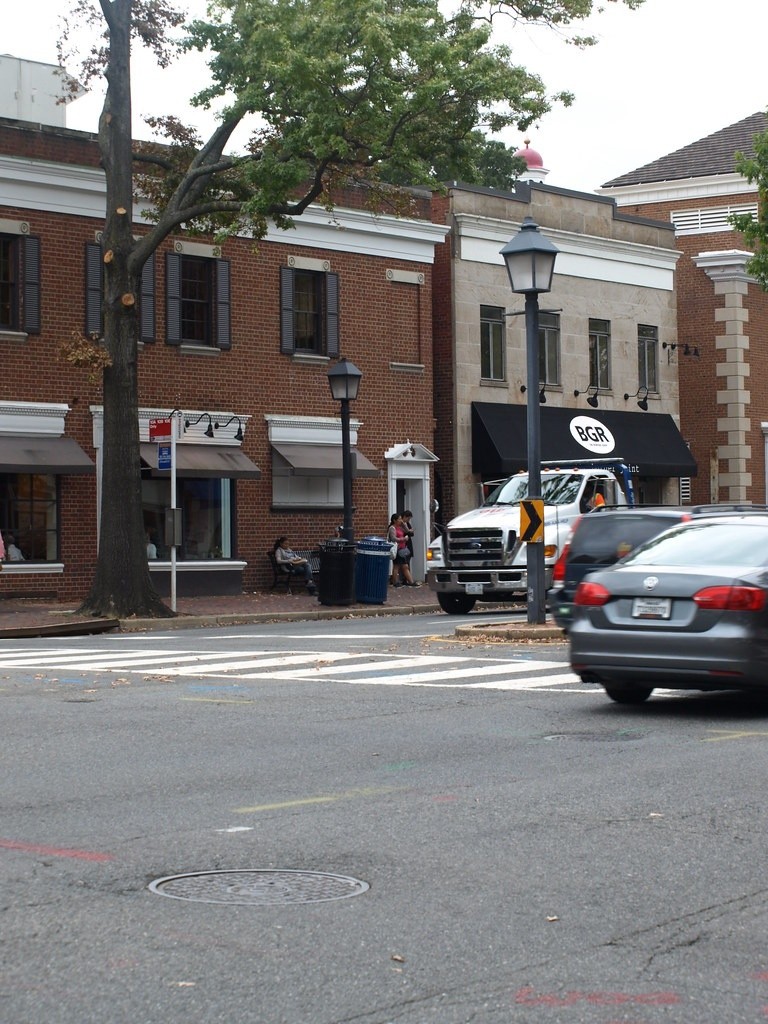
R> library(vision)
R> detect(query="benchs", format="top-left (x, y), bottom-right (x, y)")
top-left (266, 550), bottom-right (320, 593)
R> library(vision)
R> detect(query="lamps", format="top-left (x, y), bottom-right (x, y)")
top-left (662, 342), bottom-right (691, 355)
top-left (168, 408), bottom-right (187, 433)
top-left (574, 382), bottom-right (599, 408)
top-left (624, 386), bottom-right (649, 411)
top-left (521, 378), bottom-right (547, 404)
top-left (215, 416), bottom-right (244, 440)
top-left (185, 413), bottom-right (214, 437)
top-left (671, 342), bottom-right (700, 357)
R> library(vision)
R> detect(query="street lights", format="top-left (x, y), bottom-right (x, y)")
top-left (322, 358), bottom-right (365, 602)
top-left (499, 213), bottom-right (562, 625)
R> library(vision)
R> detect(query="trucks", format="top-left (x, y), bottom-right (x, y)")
top-left (425, 458), bottom-right (636, 614)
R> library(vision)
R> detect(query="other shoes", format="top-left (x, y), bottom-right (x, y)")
top-left (393, 582), bottom-right (404, 589)
top-left (408, 581), bottom-right (421, 588)
top-left (306, 584), bottom-right (316, 588)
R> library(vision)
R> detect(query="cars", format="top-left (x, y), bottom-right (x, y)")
top-left (567, 515), bottom-right (767, 710)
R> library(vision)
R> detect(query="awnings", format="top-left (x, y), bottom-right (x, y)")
top-left (0, 436), bottom-right (97, 474)
top-left (139, 443), bottom-right (262, 482)
top-left (469, 401), bottom-right (698, 478)
top-left (273, 442), bottom-right (379, 478)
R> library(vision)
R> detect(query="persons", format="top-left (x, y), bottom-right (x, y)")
top-left (145, 530), bottom-right (157, 559)
top-left (0, 530), bottom-right (25, 561)
top-left (384, 508), bottom-right (422, 588)
top-left (275, 536), bottom-right (319, 595)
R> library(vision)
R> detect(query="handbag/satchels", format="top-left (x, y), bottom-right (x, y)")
top-left (386, 526), bottom-right (398, 560)
top-left (398, 547), bottom-right (411, 559)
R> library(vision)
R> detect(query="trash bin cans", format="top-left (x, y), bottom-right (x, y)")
top-left (316, 537), bottom-right (357, 607)
top-left (355, 535), bottom-right (394, 605)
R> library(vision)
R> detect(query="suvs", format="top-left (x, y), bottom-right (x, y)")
top-left (550, 506), bottom-right (766, 627)
